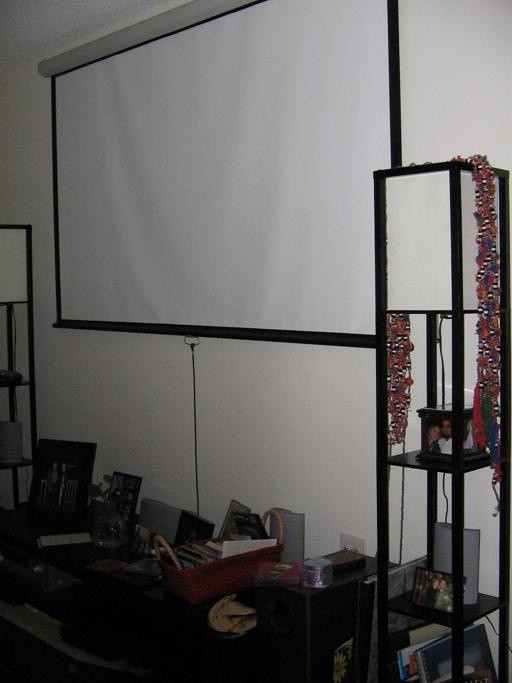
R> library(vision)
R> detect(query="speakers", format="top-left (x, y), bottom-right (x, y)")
top-left (254, 550), bottom-right (397, 683)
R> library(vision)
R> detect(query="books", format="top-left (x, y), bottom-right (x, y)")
top-left (161, 539), bottom-right (232, 568)
top-left (395, 624), bottom-right (484, 683)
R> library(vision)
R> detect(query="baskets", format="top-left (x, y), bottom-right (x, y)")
top-left (153, 509), bottom-right (284, 604)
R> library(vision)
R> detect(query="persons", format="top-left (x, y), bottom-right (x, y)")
top-left (422, 577), bottom-right (452, 612)
top-left (425, 419), bottom-right (453, 455)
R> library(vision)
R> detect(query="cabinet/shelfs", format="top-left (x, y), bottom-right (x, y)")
top-left (373, 161), bottom-right (511, 683)
top-left (0, 223), bottom-right (38, 508)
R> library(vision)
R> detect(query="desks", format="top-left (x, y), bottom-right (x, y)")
top-left (0, 502), bottom-right (171, 609)
